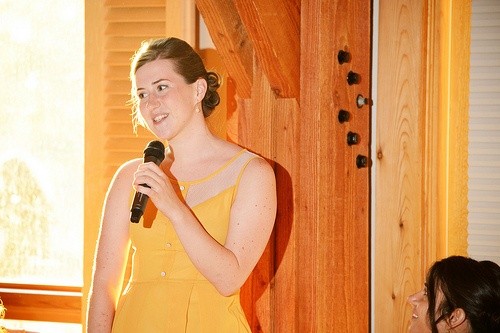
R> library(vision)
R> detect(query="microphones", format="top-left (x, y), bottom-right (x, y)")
top-left (130, 140), bottom-right (165, 223)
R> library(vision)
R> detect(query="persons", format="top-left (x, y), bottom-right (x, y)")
top-left (407, 255), bottom-right (500, 333)
top-left (85, 37), bottom-right (277, 333)
